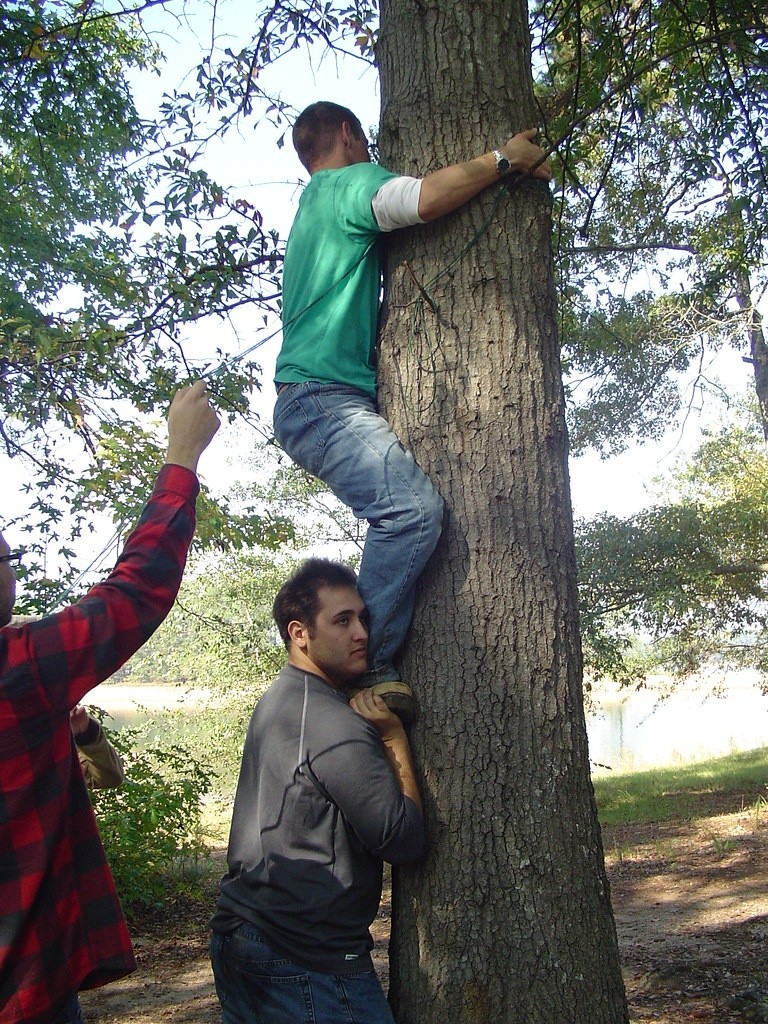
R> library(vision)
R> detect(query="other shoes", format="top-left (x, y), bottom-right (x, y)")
top-left (346, 680), bottom-right (414, 724)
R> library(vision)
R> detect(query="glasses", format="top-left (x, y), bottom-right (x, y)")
top-left (0, 552), bottom-right (22, 568)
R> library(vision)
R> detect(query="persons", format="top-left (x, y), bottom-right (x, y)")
top-left (273, 101), bottom-right (553, 713)
top-left (0, 378), bottom-right (221, 1024)
top-left (209, 558), bottom-right (427, 1024)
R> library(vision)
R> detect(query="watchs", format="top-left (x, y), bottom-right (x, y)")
top-left (491, 149), bottom-right (511, 178)
